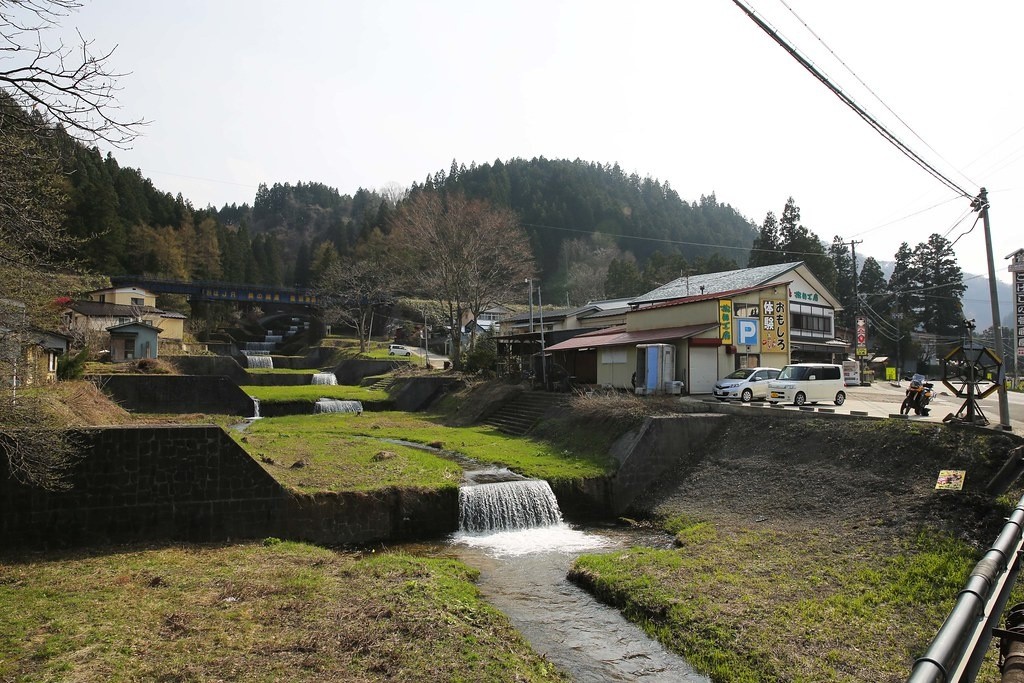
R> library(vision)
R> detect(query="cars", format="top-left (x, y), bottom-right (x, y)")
top-left (712, 367), bottom-right (788, 403)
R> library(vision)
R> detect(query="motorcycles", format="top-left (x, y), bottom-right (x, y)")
top-left (899, 373), bottom-right (935, 416)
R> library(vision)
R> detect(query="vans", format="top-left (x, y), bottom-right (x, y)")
top-left (388, 345), bottom-right (412, 356)
top-left (765, 363), bottom-right (846, 406)
